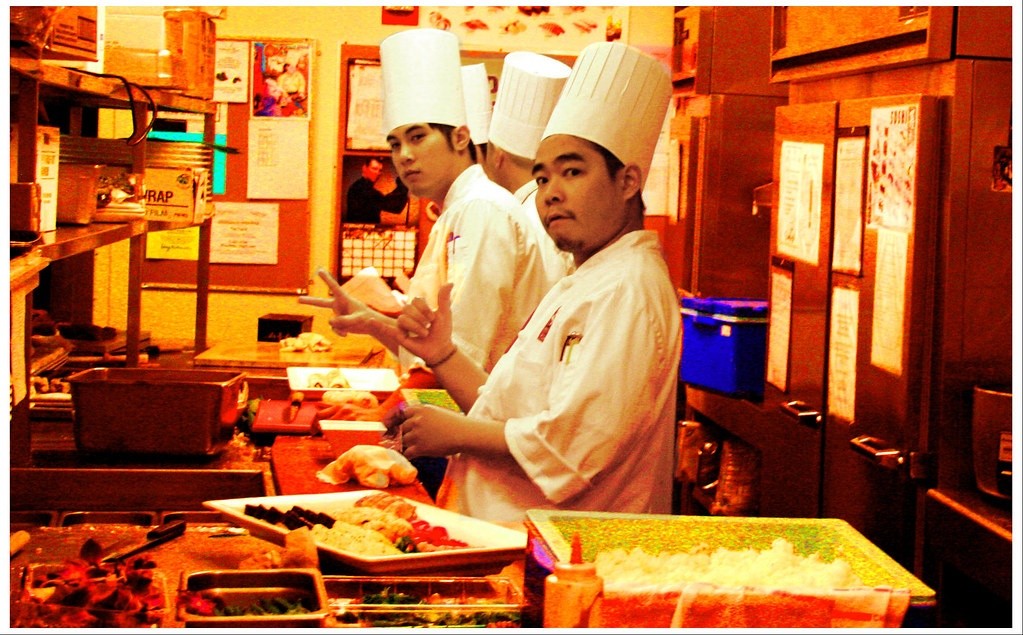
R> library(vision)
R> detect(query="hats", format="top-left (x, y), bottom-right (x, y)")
top-left (460, 63), bottom-right (493, 145)
top-left (541, 41), bottom-right (672, 192)
top-left (489, 51), bottom-right (571, 161)
top-left (379, 28), bottom-right (467, 139)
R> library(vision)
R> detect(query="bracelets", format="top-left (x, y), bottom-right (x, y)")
top-left (425, 344), bottom-right (458, 368)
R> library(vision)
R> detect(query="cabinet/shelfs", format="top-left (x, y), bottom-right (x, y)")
top-left (664, 6), bottom-right (1012, 628)
top-left (10, 47), bottom-right (217, 470)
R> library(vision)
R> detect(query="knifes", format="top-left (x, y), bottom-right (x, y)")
top-left (99, 521), bottom-right (187, 561)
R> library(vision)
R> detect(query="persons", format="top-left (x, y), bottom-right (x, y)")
top-left (347, 157), bottom-right (408, 224)
top-left (298, 27), bottom-right (579, 375)
top-left (395, 42), bottom-right (685, 532)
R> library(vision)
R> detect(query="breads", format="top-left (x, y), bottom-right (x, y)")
top-left (310, 369), bottom-right (378, 409)
top-left (279, 333), bottom-right (334, 352)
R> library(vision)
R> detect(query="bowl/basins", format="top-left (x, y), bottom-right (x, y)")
top-left (60, 367), bottom-right (248, 455)
top-left (286, 366), bottom-right (400, 399)
top-left (175, 567), bottom-right (331, 628)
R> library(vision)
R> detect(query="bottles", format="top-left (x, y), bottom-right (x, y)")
top-left (543, 533), bottom-right (604, 628)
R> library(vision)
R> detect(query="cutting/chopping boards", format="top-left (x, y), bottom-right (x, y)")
top-left (270, 435), bottom-right (434, 507)
top-left (194, 338), bottom-right (375, 370)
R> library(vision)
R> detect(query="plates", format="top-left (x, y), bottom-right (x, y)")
top-left (319, 419), bottom-right (388, 447)
top-left (202, 489), bottom-right (527, 574)
top-left (59, 135), bottom-right (212, 224)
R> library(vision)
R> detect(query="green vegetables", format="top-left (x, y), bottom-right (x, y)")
top-left (212, 587), bottom-right (527, 628)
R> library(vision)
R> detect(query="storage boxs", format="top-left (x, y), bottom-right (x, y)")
top-left (70, 6), bottom-right (190, 91)
top-left (682, 296), bottom-right (767, 393)
top-left (158, 6), bottom-right (216, 99)
top-left (523, 509), bottom-right (936, 627)
top-left (62, 367), bottom-right (246, 456)
